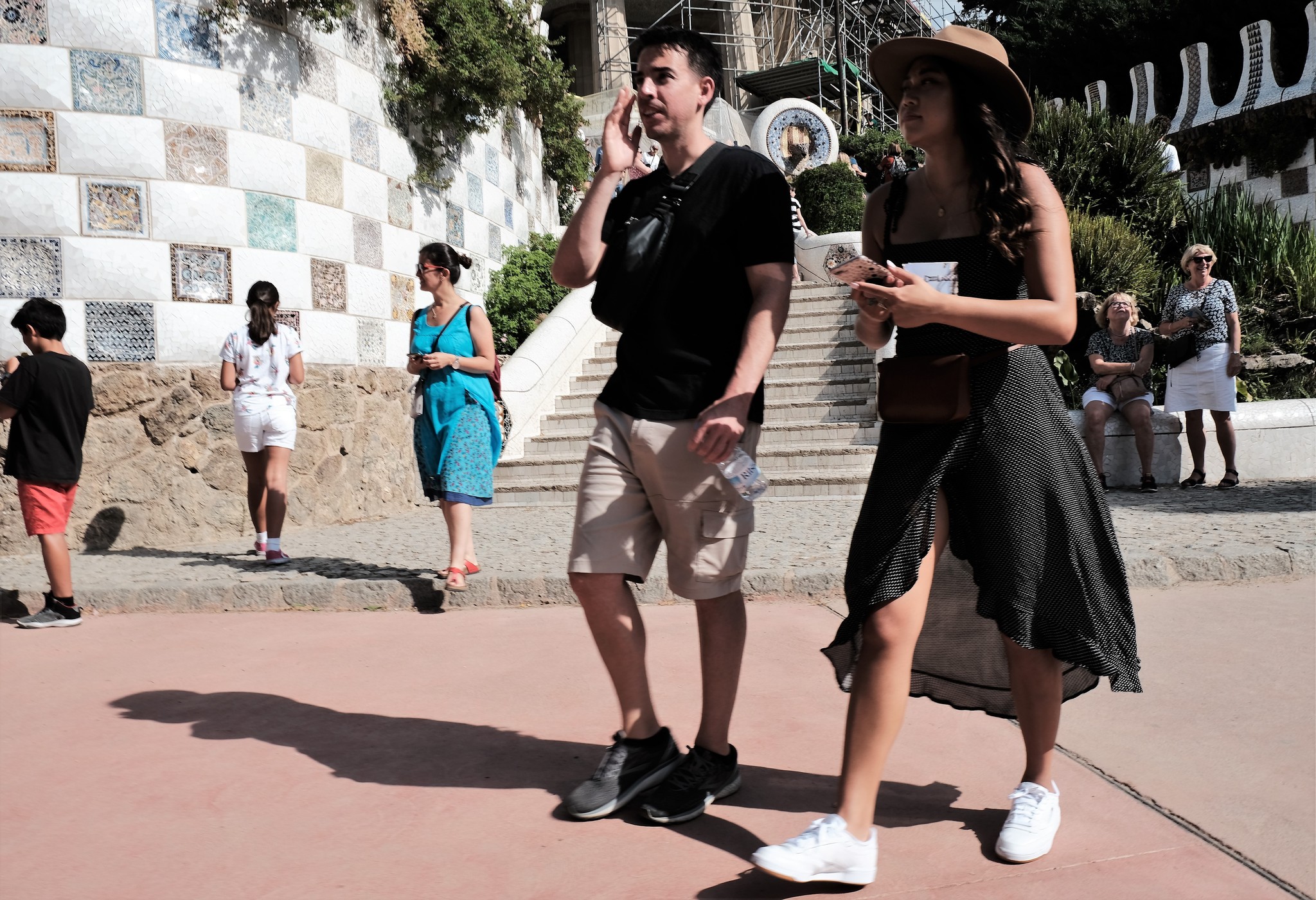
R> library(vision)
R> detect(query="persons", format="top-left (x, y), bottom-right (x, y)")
top-left (558, 119), bottom-right (659, 212)
top-left (1159, 244), bottom-right (1242, 489)
top-left (407, 242), bottom-right (502, 589)
top-left (1083, 293), bottom-right (1157, 492)
top-left (837, 141), bottom-right (919, 199)
top-left (750, 24), bottom-right (1142, 886)
top-left (219, 281), bottom-right (304, 564)
top-left (550, 28), bottom-right (795, 825)
top-left (787, 183), bottom-right (812, 282)
top-left (0, 297), bottom-right (94, 627)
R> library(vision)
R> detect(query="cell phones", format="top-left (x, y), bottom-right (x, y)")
top-left (831, 255), bottom-right (895, 288)
top-left (407, 352), bottom-right (425, 360)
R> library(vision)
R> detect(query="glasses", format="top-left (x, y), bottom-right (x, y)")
top-left (1105, 301), bottom-right (1133, 315)
top-left (1187, 255), bottom-right (1213, 264)
top-left (415, 263), bottom-right (446, 276)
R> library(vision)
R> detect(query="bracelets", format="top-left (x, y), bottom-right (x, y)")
top-left (1131, 362), bottom-right (1136, 372)
top-left (1168, 323), bottom-right (1172, 330)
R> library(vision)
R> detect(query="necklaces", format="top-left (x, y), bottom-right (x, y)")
top-left (1108, 327), bottom-right (1128, 336)
top-left (431, 302), bottom-right (455, 318)
top-left (923, 166), bottom-right (965, 216)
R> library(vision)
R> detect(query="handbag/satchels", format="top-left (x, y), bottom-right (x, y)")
top-left (876, 354), bottom-right (970, 422)
top-left (1109, 372), bottom-right (1150, 404)
top-left (588, 141), bottom-right (731, 334)
top-left (408, 381), bottom-right (423, 417)
top-left (1166, 332), bottom-right (1200, 369)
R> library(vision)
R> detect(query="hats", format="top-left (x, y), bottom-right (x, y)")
top-left (868, 23), bottom-right (1034, 148)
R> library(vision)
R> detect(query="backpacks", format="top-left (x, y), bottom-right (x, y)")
top-left (413, 304), bottom-right (501, 402)
top-left (883, 155), bottom-right (907, 179)
top-left (837, 152), bottom-right (857, 178)
top-left (906, 161), bottom-right (920, 176)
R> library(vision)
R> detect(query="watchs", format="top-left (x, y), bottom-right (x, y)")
top-left (1231, 351), bottom-right (1239, 354)
top-left (451, 355), bottom-right (461, 370)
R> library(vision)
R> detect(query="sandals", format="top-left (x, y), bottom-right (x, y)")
top-left (1217, 468), bottom-right (1239, 489)
top-left (1180, 468), bottom-right (1207, 488)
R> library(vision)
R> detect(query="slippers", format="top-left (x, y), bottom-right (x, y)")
top-left (438, 559), bottom-right (482, 580)
top-left (445, 567), bottom-right (467, 592)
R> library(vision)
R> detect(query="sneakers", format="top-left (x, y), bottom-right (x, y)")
top-left (16, 592), bottom-right (84, 629)
top-left (639, 743), bottom-right (743, 823)
top-left (1098, 472), bottom-right (1111, 492)
top-left (254, 540), bottom-right (267, 556)
top-left (1139, 473), bottom-right (1158, 492)
top-left (752, 813), bottom-right (878, 886)
top-left (265, 549), bottom-right (291, 564)
top-left (564, 726), bottom-right (686, 822)
top-left (995, 780), bottom-right (1062, 863)
